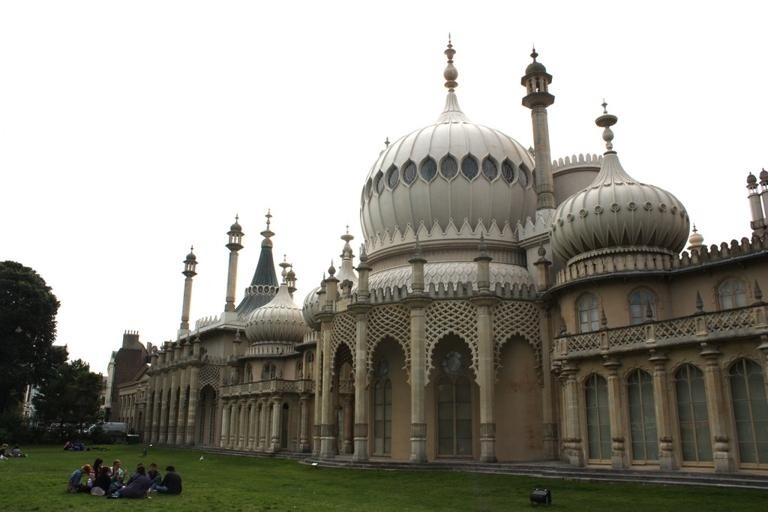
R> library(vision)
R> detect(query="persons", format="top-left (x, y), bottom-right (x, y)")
top-left (0, 443), bottom-right (27, 458)
top-left (63, 440), bottom-right (85, 451)
top-left (67, 458), bottom-right (182, 499)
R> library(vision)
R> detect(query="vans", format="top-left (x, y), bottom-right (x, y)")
top-left (87, 422), bottom-right (127, 434)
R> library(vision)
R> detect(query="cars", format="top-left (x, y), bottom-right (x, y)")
top-left (76, 422), bottom-right (85, 430)
top-left (49, 423), bottom-right (71, 431)
